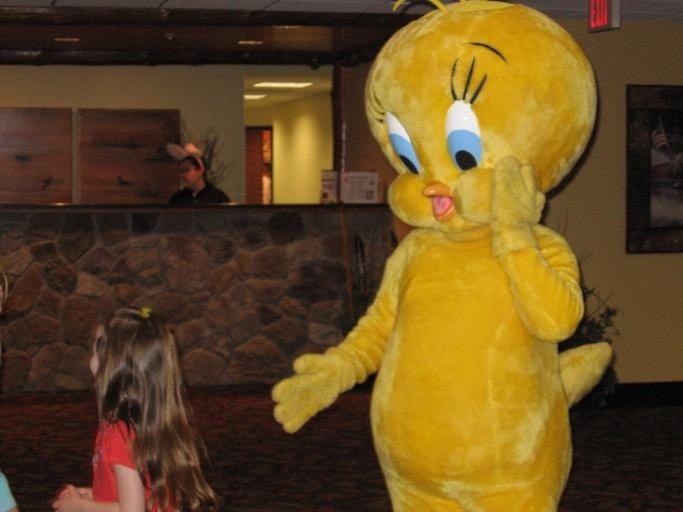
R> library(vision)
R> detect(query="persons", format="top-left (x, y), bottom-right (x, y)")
top-left (164, 151), bottom-right (235, 203)
top-left (46, 304), bottom-right (220, 511)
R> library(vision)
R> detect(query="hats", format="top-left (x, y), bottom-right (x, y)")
top-left (164, 140), bottom-right (200, 161)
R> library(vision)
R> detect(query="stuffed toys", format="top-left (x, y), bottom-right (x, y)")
top-left (270, 0), bottom-right (614, 512)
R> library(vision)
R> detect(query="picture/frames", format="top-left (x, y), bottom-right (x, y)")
top-left (626, 84), bottom-right (683, 254)
